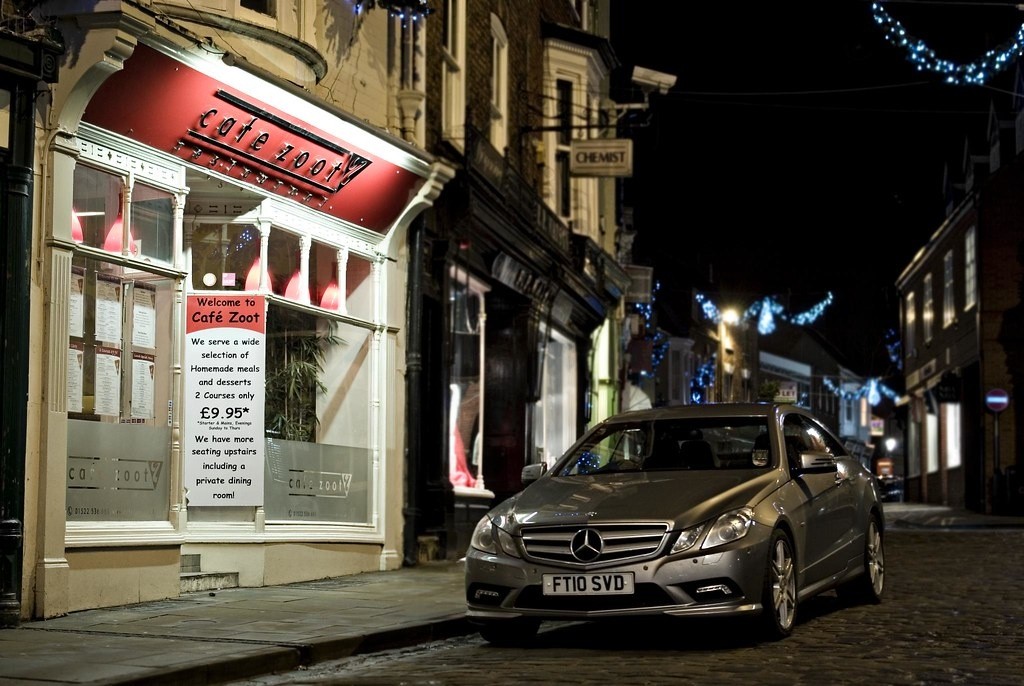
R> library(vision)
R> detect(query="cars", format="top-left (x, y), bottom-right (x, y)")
top-left (463, 402), bottom-right (888, 642)
top-left (877, 475), bottom-right (903, 501)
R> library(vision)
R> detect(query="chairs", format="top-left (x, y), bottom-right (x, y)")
top-left (681, 440), bottom-right (714, 471)
top-left (750, 433), bottom-right (797, 463)
top-left (642, 437), bottom-right (683, 469)
